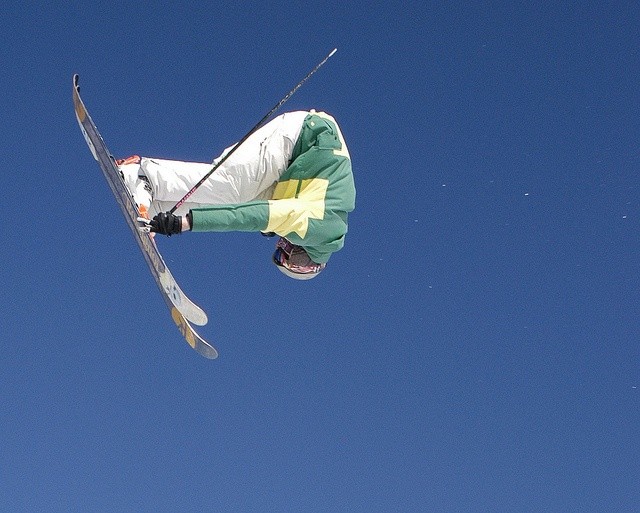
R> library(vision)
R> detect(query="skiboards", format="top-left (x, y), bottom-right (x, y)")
top-left (72, 73), bottom-right (218, 360)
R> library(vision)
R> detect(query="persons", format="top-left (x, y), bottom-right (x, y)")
top-left (113, 110), bottom-right (356, 280)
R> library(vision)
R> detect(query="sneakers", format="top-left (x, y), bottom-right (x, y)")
top-left (119, 153), bottom-right (153, 209)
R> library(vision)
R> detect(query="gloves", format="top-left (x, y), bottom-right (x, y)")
top-left (138, 211), bottom-right (182, 236)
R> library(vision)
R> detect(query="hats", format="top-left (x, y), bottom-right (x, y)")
top-left (272, 257), bottom-right (321, 280)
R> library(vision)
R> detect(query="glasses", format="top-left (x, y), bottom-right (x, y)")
top-left (273, 248), bottom-right (284, 263)
